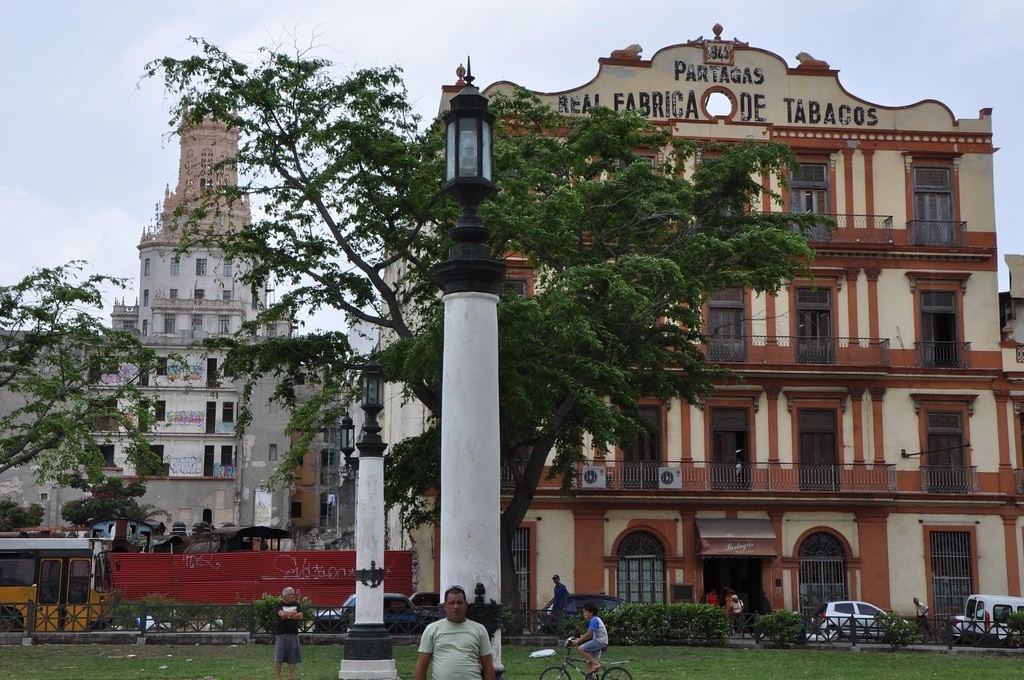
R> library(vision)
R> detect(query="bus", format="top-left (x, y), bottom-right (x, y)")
top-left (0, 537), bottom-right (122, 633)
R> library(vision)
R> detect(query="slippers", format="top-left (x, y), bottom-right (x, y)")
top-left (588, 666), bottom-right (601, 674)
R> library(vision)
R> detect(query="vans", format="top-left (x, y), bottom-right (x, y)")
top-left (965, 593), bottom-right (1024, 639)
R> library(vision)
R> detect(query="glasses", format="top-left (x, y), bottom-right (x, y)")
top-left (444, 586), bottom-right (466, 601)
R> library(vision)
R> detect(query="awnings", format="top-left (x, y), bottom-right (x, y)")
top-left (694, 517), bottom-right (778, 561)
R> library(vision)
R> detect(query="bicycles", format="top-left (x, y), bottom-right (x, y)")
top-left (540, 635), bottom-right (634, 680)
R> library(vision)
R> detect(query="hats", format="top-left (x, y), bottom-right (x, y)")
top-left (552, 575), bottom-right (559, 579)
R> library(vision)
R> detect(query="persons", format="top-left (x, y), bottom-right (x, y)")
top-left (551, 574), bottom-right (570, 621)
top-left (274, 587), bottom-right (304, 680)
top-left (913, 598), bottom-right (935, 641)
top-left (706, 587), bottom-right (744, 636)
top-left (413, 587), bottom-right (496, 680)
top-left (571, 602), bottom-right (609, 680)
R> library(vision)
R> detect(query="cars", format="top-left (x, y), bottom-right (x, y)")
top-left (810, 602), bottom-right (909, 642)
top-left (538, 592), bottom-right (631, 619)
top-left (409, 591), bottom-right (440, 620)
top-left (315, 593), bottom-right (422, 634)
top-left (949, 615), bottom-right (965, 637)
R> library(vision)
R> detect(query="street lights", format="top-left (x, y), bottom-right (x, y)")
top-left (432, 49), bottom-right (505, 679)
top-left (338, 350), bottom-right (400, 679)
